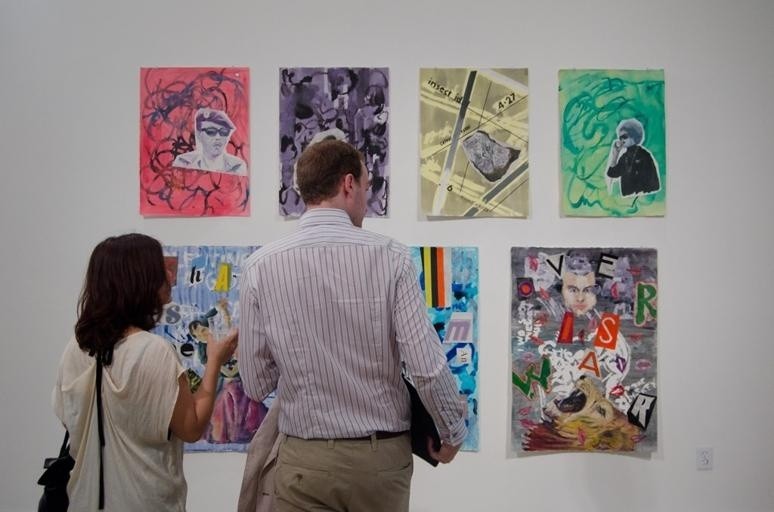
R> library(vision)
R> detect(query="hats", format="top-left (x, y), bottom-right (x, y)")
top-left (195, 108), bottom-right (236, 130)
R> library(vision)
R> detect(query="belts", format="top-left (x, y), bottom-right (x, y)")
top-left (287, 428), bottom-right (410, 440)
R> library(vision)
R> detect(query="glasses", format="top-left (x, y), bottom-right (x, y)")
top-left (201, 127), bottom-right (230, 138)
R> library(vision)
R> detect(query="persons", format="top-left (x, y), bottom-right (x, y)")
top-left (234, 138), bottom-right (468, 510)
top-left (50, 232), bottom-right (239, 509)
top-left (559, 268), bottom-right (599, 317)
top-left (173, 108), bottom-right (250, 178)
top-left (601, 114), bottom-right (662, 201)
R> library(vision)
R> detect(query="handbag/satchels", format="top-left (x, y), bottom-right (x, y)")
top-left (36, 453), bottom-right (76, 512)
